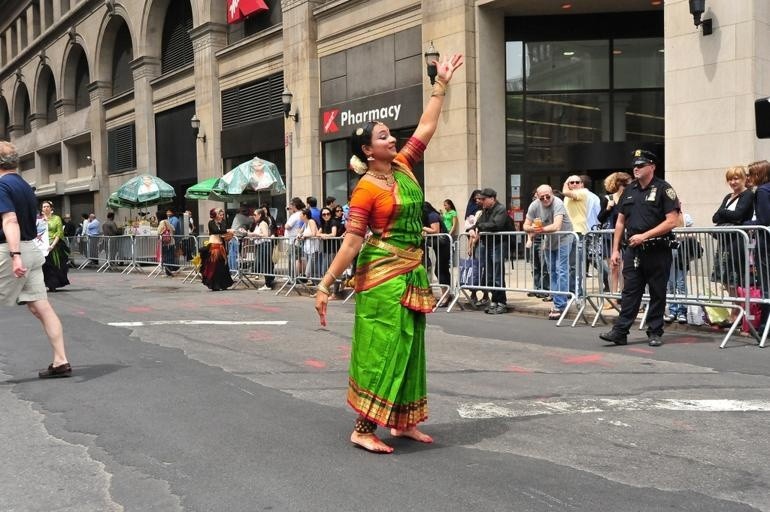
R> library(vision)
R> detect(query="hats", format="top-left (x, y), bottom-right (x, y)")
top-left (631, 149), bottom-right (658, 167)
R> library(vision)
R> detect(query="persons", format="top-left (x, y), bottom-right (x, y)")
top-left (0, 141), bottom-right (72, 378)
top-left (314, 52), bottom-right (464, 455)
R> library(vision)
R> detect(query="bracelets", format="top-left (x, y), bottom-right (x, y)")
top-left (432, 77), bottom-right (448, 97)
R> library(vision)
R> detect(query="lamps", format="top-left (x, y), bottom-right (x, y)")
top-left (281, 82), bottom-right (299, 124)
top-left (688, 1), bottom-right (713, 36)
top-left (424, 40), bottom-right (442, 87)
top-left (191, 112), bottom-right (208, 145)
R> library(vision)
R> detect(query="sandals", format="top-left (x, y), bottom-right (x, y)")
top-left (526, 291), bottom-right (563, 320)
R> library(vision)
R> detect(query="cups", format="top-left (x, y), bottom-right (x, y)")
top-left (533, 217), bottom-right (543, 231)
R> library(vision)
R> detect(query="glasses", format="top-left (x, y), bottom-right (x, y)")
top-left (633, 164), bottom-right (653, 169)
top-left (318, 176), bottom-right (583, 221)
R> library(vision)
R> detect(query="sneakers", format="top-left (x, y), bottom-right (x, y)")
top-left (600, 301), bottom-right (688, 326)
top-left (598, 324), bottom-right (632, 347)
top-left (434, 294), bottom-right (509, 316)
top-left (644, 329), bottom-right (666, 347)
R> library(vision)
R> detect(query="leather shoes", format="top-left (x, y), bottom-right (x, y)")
top-left (38, 361), bottom-right (74, 380)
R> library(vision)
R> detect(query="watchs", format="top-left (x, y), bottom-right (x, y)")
top-left (9, 252), bottom-right (21, 258)
